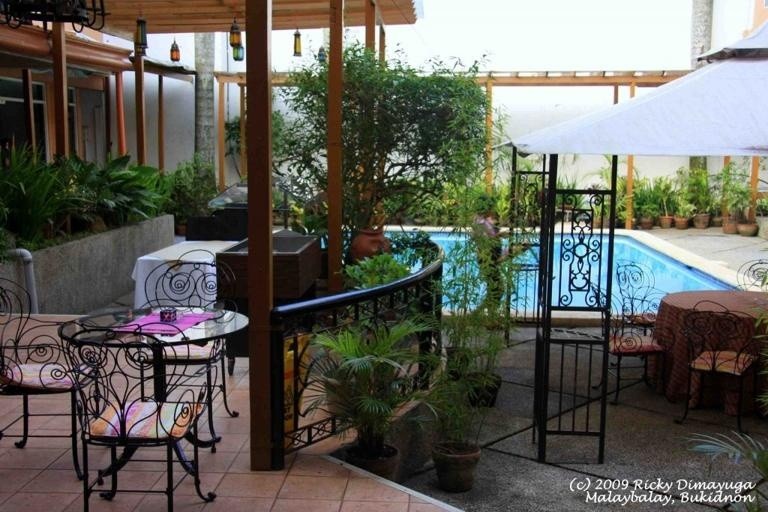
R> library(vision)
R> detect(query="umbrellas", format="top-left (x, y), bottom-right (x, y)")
top-left (501, 18), bottom-right (768, 163)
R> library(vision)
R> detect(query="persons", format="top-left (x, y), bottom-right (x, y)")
top-left (470, 194), bottom-right (530, 316)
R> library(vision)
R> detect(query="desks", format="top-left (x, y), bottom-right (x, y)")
top-left (131, 240), bottom-right (238, 312)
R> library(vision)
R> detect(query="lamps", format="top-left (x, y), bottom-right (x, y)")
top-left (232, 45), bottom-right (245, 62)
top-left (292, 27), bottom-right (303, 57)
top-left (170, 32), bottom-right (180, 61)
top-left (132, 1), bottom-right (148, 58)
top-left (229, 19), bottom-right (241, 45)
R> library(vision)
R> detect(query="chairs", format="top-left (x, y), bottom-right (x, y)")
top-left (588, 257), bottom-right (768, 433)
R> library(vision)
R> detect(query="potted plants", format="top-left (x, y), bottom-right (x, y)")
top-left (591, 157), bottom-right (760, 235)
top-left (0, 137), bottom-right (215, 317)
